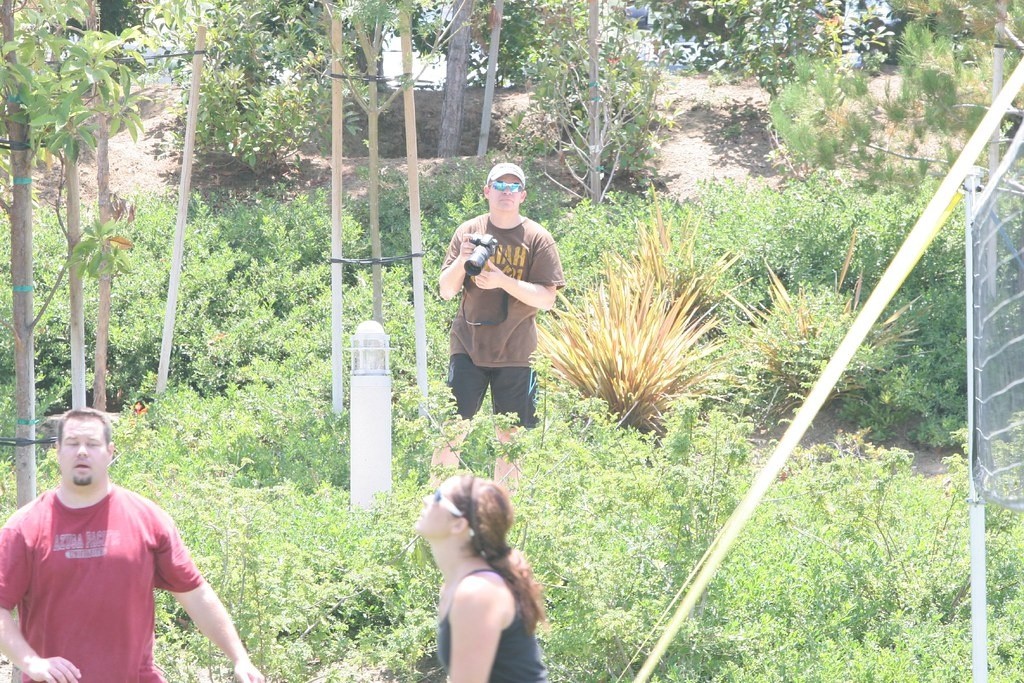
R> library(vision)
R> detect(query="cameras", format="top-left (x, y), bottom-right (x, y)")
top-left (464, 232), bottom-right (499, 276)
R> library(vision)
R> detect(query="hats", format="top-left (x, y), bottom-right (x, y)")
top-left (487, 162), bottom-right (525, 187)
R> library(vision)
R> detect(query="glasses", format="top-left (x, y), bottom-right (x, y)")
top-left (434, 489), bottom-right (463, 517)
top-left (490, 181), bottom-right (524, 192)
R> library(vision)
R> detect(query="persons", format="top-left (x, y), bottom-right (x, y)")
top-left (0, 408), bottom-right (264, 683)
top-left (431, 163), bottom-right (566, 495)
top-left (414, 475), bottom-right (549, 683)
